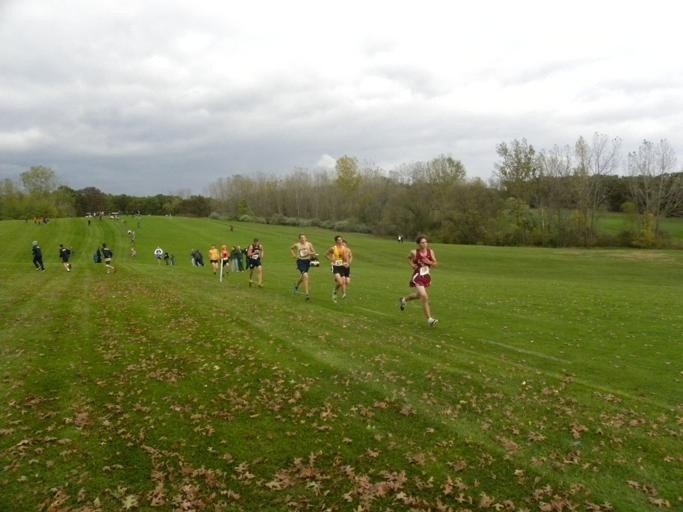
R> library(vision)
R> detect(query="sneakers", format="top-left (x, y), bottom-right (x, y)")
top-left (213, 268), bottom-right (264, 289)
top-left (399, 296), bottom-right (406, 311)
top-left (430, 319), bottom-right (439, 328)
top-left (292, 285), bottom-right (349, 304)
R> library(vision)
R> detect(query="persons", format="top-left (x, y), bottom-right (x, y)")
top-left (101, 243), bottom-right (114, 273)
top-left (24, 215), bottom-right (48, 224)
top-left (58, 244), bottom-right (71, 272)
top-left (323, 234), bottom-right (347, 304)
top-left (153, 244), bottom-right (251, 276)
top-left (289, 233), bottom-right (315, 301)
top-left (31, 241), bottom-right (47, 271)
top-left (246, 238), bottom-right (264, 288)
top-left (335, 239), bottom-right (351, 298)
top-left (311, 256), bottom-right (314, 260)
top-left (87, 209), bottom-right (143, 228)
top-left (398, 233), bottom-right (438, 327)
top-left (314, 257), bottom-right (317, 260)
